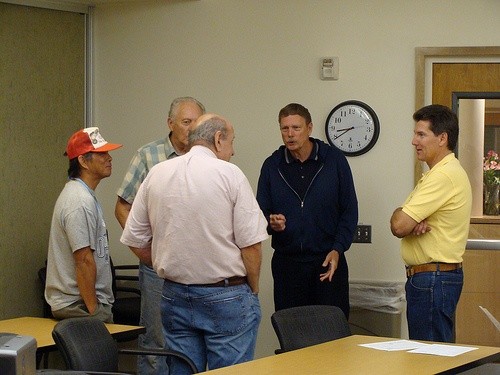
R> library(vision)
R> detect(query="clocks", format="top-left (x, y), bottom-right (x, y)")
top-left (325, 100), bottom-right (380, 157)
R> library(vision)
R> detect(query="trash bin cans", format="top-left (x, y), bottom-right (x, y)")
top-left (349, 279), bottom-right (406, 338)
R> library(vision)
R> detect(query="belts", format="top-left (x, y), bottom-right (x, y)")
top-left (405, 262), bottom-right (462, 277)
top-left (164, 276), bottom-right (247, 287)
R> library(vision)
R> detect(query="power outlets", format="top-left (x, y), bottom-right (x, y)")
top-left (352, 225), bottom-right (371, 243)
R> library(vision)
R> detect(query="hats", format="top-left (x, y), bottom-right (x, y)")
top-left (66, 126), bottom-right (122, 161)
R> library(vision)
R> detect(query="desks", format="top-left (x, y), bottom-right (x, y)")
top-left (193, 335), bottom-right (500, 375)
top-left (0, 317), bottom-right (147, 369)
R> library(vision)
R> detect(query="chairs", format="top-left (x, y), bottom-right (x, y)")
top-left (52, 317), bottom-right (199, 375)
top-left (108, 255), bottom-right (142, 325)
top-left (271, 305), bottom-right (351, 355)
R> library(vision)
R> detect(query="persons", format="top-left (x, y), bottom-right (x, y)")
top-left (120, 114), bottom-right (269, 375)
top-left (114, 97), bottom-right (207, 375)
top-left (256, 102), bottom-right (359, 319)
top-left (389, 105), bottom-right (472, 344)
top-left (44, 126), bottom-right (124, 324)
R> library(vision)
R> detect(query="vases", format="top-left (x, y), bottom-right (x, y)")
top-left (484, 185), bottom-right (500, 214)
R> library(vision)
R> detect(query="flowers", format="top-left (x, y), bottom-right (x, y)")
top-left (483, 151), bottom-right (500, 214)
